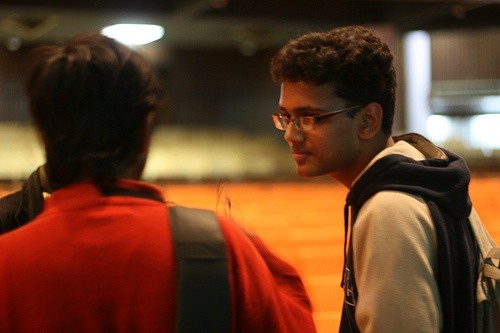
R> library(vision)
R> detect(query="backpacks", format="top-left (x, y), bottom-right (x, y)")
top-left (477, 255), bottom-right (500, 333)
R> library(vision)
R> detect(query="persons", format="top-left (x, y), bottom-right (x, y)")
top-left (271, 22), bottom-right (500, 332)
top-left (0, 32), bottom-right (319, 333)
top-left (0, 160), bottom-right (53, 237)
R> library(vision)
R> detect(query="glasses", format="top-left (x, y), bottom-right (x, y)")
top-left (272, 103), bottom-right (362, 130)
